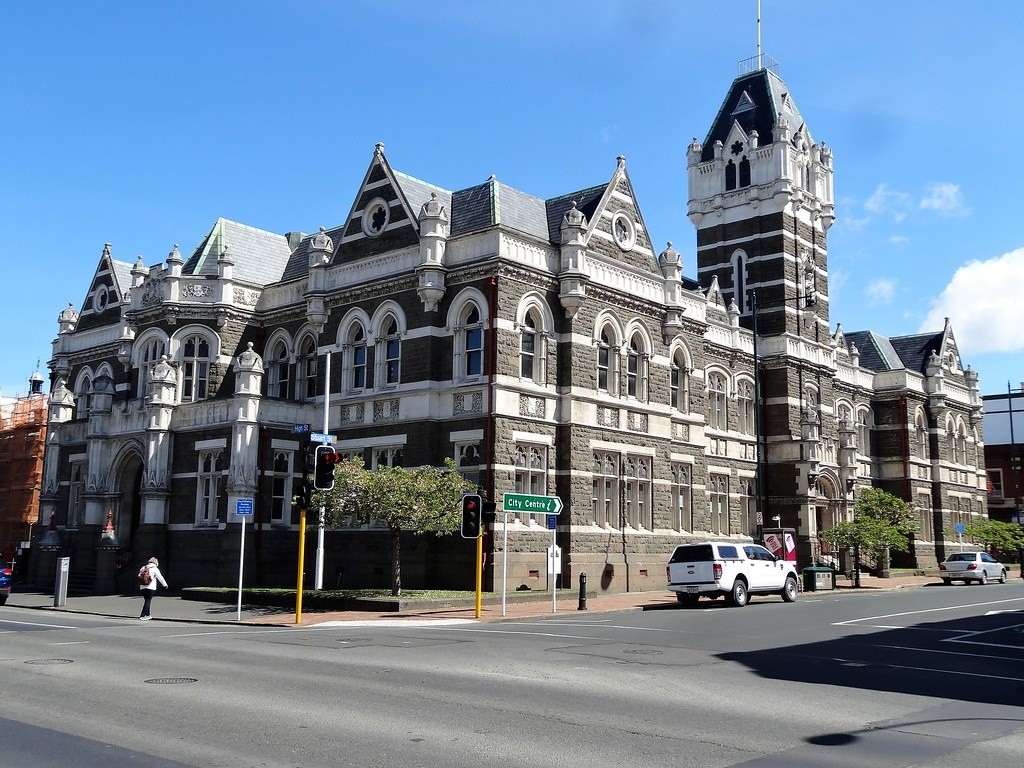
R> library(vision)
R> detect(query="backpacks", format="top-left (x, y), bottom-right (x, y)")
top-left (138, 570), bottom-right (152, 587)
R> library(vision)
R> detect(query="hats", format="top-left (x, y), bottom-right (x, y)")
top-left (147, 557), bottom-right (159, 566)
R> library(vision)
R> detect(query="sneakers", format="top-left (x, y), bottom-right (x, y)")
top-left (139, 617), bottom-right (145, 620)
top-left (146, 616), bottom-right (152, 619)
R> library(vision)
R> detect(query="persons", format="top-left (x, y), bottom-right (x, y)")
top-left (138, 557), bottom-right (168, 622)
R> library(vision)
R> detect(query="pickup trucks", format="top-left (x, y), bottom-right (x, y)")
top-left (666, 542), bottom-right (799, 607)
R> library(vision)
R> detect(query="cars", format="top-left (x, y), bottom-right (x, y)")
top-left (939, 552), bottom-right (1007, 585)
top-left (0, 553), bottom-right (12, 605)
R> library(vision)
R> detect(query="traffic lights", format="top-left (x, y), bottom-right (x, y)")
top-left (314, 445), bottom-right (343, 491)
top-left (461, 495), bottom-right (482, 539)
top-left (297, 480), bottom-right (311, 509)
